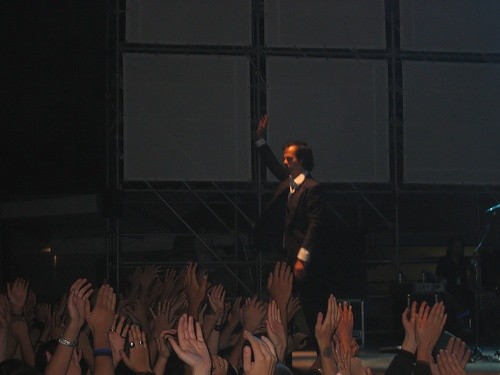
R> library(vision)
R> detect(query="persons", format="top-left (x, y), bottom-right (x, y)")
top-left (252, 113), bottom-right (325, 341)
top-left (0, 255), bottom-right (500, 375)
top-left (433, 238), bottom-right (469, 286)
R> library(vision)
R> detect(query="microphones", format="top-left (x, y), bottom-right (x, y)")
top-left (484, 203), bottom-right (500, 216)
